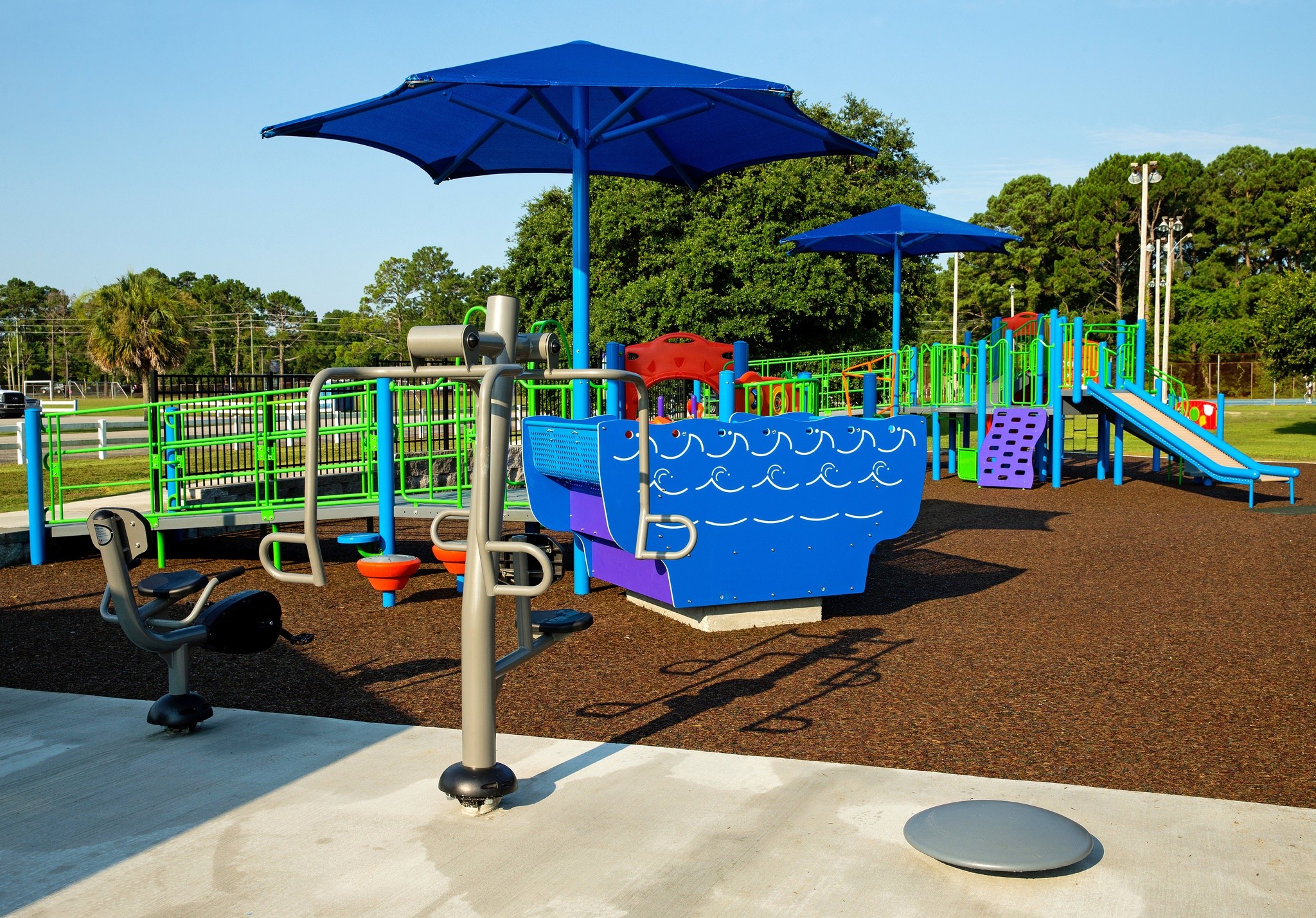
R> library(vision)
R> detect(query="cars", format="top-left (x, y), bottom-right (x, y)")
top-left (115, 383), bottom-right (142, 394)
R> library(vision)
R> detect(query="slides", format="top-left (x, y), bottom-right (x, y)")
top-left (1085, 379), bottom-right (1301, 509)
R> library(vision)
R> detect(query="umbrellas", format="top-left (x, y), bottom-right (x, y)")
top-left (260, 40), bottom-right (879, 597)
top-left (778, 204), bottom-right (1024, 416)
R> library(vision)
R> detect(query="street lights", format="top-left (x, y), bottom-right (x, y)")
top-left (1129, 160), bottom-right (1183, 404)
top-left (1009, 283), bottom-right (1015, 351)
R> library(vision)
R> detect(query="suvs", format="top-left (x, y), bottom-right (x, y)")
top-left (41, 382), bottom-right (66, 395)
